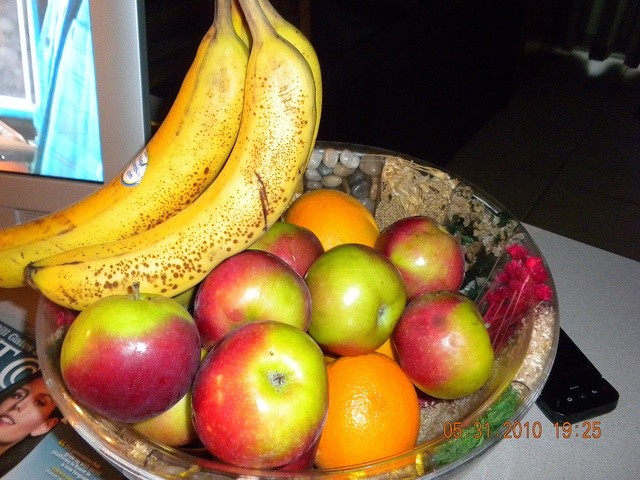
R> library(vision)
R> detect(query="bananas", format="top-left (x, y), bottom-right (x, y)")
top-left (0, 0), bottom-right (323, 311)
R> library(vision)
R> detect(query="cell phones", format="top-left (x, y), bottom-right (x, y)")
top-left (535, 326), bottom-right (619, 427)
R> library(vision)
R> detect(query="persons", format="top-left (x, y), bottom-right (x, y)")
top-left (0, 369), bottom-right (63, 479)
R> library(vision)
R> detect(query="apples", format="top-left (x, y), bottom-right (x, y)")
top-left (247, 220), bottom-right (325, 278)
top-left (192, 320), bottom-right (329, 468)
top-left (374, 216), bottom-right (466, 304)
top-left (304, 243), bottom-right (406, 357)
top-left (391, 289), bottom-right (496, 400)
top-left (60, 282), bottom-right (202, 424)
top-left (131, 346), bottom-right (208, 447)
top-left (194, 249), bottom-right (312, 353)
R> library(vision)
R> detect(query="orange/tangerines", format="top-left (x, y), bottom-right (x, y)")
top-left (313, 352), bottom-right (421, 470)
top-left (285, 188), bottom-right (380, 253)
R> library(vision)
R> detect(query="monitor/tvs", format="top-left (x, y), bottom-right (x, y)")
top-left (0, 0), bottom-right (153, 342)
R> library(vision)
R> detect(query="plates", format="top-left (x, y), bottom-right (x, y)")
top-left (34, 140), bottom-right (560, 480)
